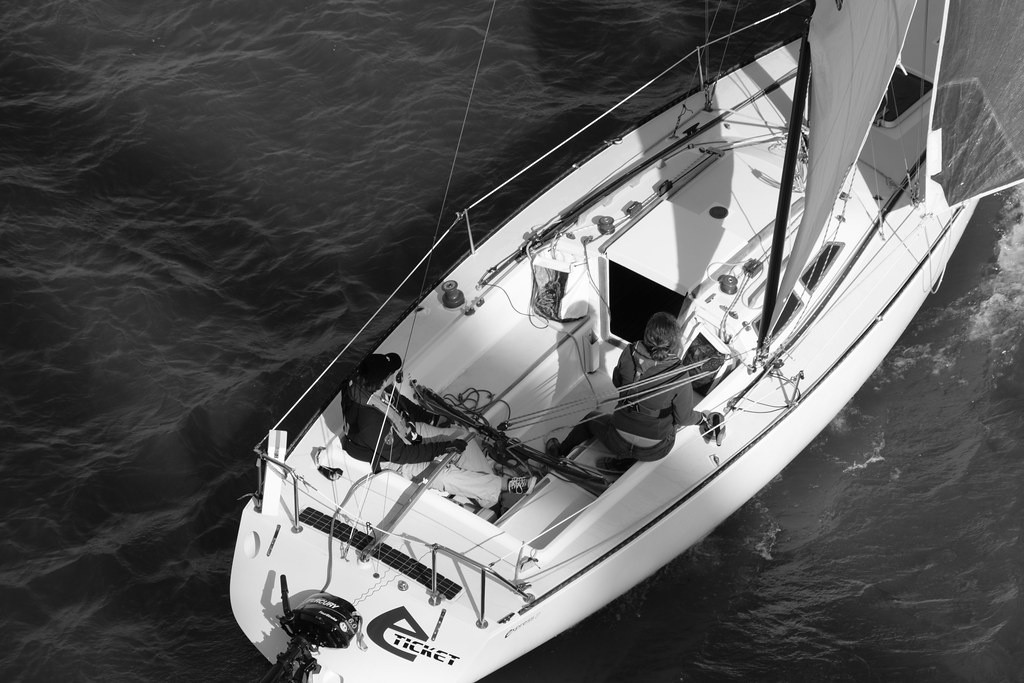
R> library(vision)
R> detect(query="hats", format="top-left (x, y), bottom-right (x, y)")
top-left (359, 353), bottom-right (402, 381)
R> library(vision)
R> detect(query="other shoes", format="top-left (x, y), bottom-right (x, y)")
top-left (596, 458), bottom-right (637, 474)
top-left (546, 437), bottom-right (567, 465)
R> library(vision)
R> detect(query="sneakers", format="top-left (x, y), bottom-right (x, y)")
top-left (507, 475), bottom-right (536, 495)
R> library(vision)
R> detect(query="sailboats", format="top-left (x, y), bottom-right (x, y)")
top-left (224, 0), bottom-right (1023, 682)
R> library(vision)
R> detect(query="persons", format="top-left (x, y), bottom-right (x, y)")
top-left (340, 353), bottom-right (537, 517)
top-left (546, 312), bottom-right (726, 476)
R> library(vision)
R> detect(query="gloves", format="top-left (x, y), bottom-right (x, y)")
top-left (431, 413), bottom-right (455, 429)
top-left (445, 438), bottom-right (469, 454)
